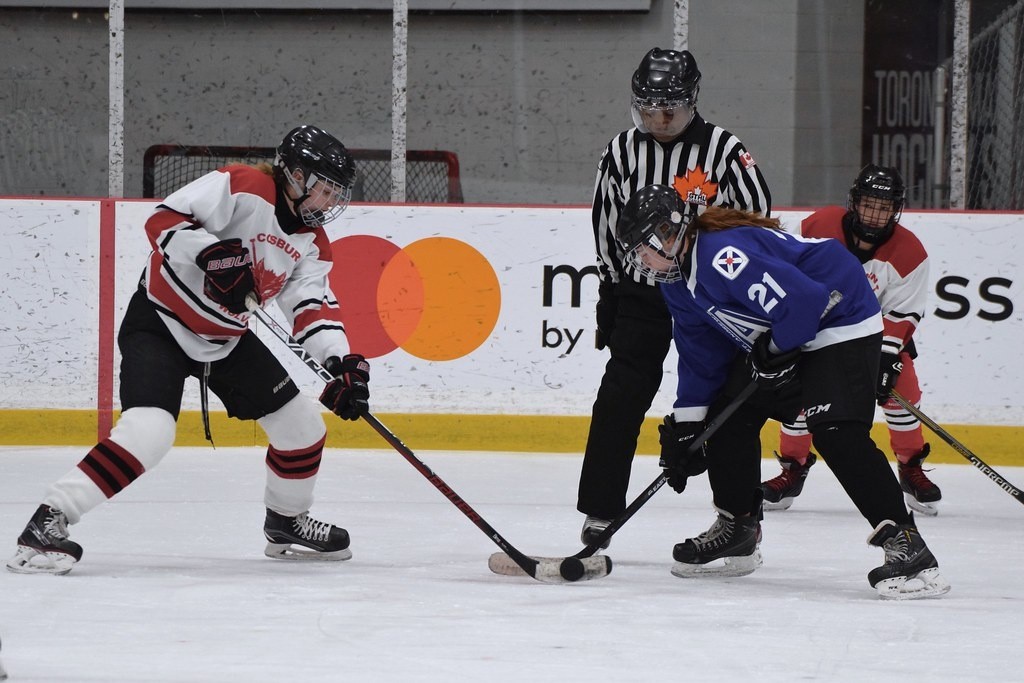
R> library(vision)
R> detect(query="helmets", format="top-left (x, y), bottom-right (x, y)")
top-left (615, 184), bottom-right (693, 284)
top-left (630, 47), bottom-right (702, 136)
top-left (846, 163), bottom-right (905, 245)
top-left (273, 123), bottom-right (359, 228)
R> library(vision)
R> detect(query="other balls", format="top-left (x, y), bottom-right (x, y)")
top-left (558, 557), bottom-right (586, 583)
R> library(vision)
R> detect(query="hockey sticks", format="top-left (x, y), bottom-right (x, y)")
top-left (888, 388), bottom-right (1024, 506)
top-left (244, 294), bottom-right (615, 584)
top-left (485, 285), bottom-right (845, 579)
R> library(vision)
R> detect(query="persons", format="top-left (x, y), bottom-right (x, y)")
top-left (577, 47), bottom-right (772, 550)
top-left (760, 162), bottom-right (942, 516)
top-left (615, 184), bottom-right (952, 601)
top-left (4, 124), bottom-right (371, 578)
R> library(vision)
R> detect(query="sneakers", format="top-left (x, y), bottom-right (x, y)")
top-left (866, 509), bottom-right (952, 598)
top-left (760, 449), bottom-right (818, 510)
top-left (263, 505), bottom-right (352, 562)
top-left (7, 505), bottom-right (82, 576)
top-left (672, 489), bottom-right (763, 577)
top-left (897, 443), bottom-right (944, 516)
top-left (581, 514), bottom-right (614, 556)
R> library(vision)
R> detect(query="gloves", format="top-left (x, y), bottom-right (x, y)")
top-left (873, 352), bottom-right (901, 406)
top-left (195, 238), bottom-right (261, 314)
top-left (320, 353), bottom-right (370, 421)
top-left (738, 330), bottom-right (801, 413)
top-left (595, 279), bottom-right (616, 350)
top-left (659, 412), bottom-right (687, 493)
top-left (664, 419), bottom-right (711, 476)
